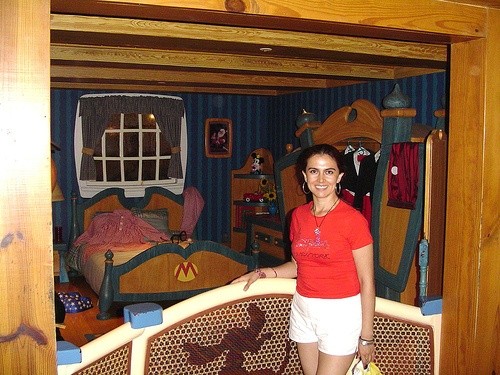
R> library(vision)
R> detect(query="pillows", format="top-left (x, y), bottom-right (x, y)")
top-left (95, 209), bottom-right (172, 242)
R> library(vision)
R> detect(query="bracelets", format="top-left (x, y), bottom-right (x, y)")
top-left (253, 268), bottom-right (267, 279)
top-left (270, 267), bottom-right (277, 278)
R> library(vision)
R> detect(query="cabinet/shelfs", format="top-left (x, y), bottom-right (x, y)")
top-left (230, 147), bottom-right (288, 262)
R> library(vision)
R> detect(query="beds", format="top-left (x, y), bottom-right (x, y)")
top-left (69, 186), bottom-right (259, 320)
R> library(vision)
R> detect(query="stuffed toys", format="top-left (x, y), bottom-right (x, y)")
top-left (250, 153), bottom-right (264, 175)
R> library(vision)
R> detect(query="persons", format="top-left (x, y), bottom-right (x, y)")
top-left (230, 144), bottom-right (376, 375)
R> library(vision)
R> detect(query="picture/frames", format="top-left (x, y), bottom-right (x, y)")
top-left (204, 118), bottom-right (233, 158)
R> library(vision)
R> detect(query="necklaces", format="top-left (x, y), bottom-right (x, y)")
top-left (313, 198), bottom-right (340, 237)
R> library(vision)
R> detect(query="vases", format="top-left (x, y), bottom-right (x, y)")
top-left (267, 202), bottom-right (278, 216)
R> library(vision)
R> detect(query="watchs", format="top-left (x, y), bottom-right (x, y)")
top-left (359, 336), bottom-right (374, 346)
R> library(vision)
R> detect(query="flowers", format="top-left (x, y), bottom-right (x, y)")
top-left (260, 177), bottom-right (277, 202)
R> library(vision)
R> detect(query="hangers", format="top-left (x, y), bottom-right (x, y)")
top-left (356, 139), bottom-right (367, 154)
top-left (343, 139), bottom-right (354, 153)
